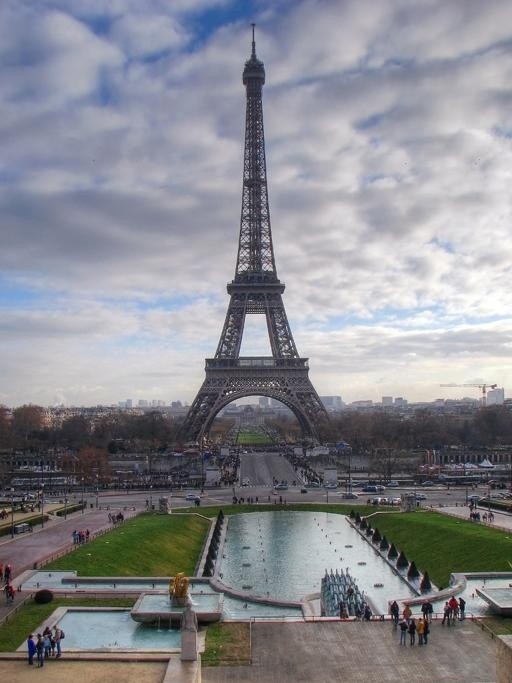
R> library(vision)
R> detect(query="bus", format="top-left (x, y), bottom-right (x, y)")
top-left (445, 476), bottom-right (480, 485)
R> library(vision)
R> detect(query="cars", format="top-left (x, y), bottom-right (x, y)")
top-left (466, 492), bottom-right (512, 500)
top-left (186, 493), bottom-right (200, 501)
top-left (342, 492), bottom-right (358, 499)
top-left (416, 493), bottom-right (426, 500)
top-left (274, 483), bottom-right (288, 490)
top-left (325, 484), bottom-right (337, 488)
top-left (488, 480), bottom-right (505, 489)
top-left (353, 483), bottom-right (364, 487)
top-left (304, 482), bottom-right (319, 488)
top-left (372, 496), bottom-right (401, 505)
top-left (362, 485), bottom-right (385, 491)
top-left (422, 481), bottom-right (433, 486)
top-left (388, 480), bottom-right (399, 487)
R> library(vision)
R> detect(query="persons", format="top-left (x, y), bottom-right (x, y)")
top-left (107, 511), bottom-right (124, 524)
top-left (28, 624), bottom-right (66, 668)
top-left (231, 493), bottom-right (283, 504)
top-left (72, 528), bottom-right (90, 544)
top-left (2, 507), bottom-right (7, 519)
top-left (269, 454), bottom-right (318, 487)
top-left (469, 510), bottom-right (495, 522)
top-left (359, 594), bottom-right (467, 648)
top-left (240, 445), bottom-right (295, 456)
top-left (126, 450), bottom-right (242, 491)
top-left (1, 563), bottom-right (17, 602)
